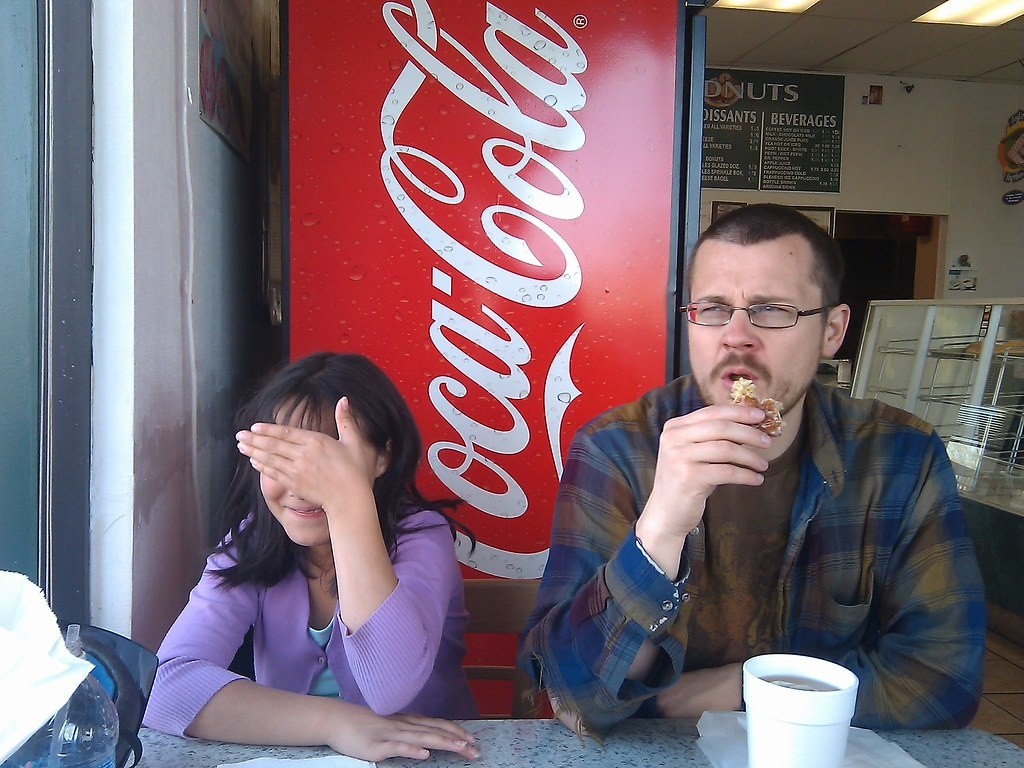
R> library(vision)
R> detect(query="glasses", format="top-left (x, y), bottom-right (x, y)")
top-left (681, 301), bottom-right (834, 330)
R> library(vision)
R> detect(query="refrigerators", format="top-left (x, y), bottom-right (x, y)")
top-left (276, 0), bottom-right (714, 720)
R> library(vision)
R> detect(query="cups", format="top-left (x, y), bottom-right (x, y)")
top-left (743, 653), bottom-right (859, 768)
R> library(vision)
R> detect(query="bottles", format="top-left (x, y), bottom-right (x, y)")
top-left (1, 623), bottom-right (118, 768)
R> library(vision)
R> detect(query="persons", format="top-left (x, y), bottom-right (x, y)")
top-left (144, 353), bottom-right (480, 761)
top-left (515, 205), bottom-right (985, 734)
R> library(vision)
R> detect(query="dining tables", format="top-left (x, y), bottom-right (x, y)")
top-left (120, 714), bottom-right (1024, 768)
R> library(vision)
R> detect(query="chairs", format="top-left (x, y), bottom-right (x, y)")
top-left (456, 580), bottom-right (541, 720)
